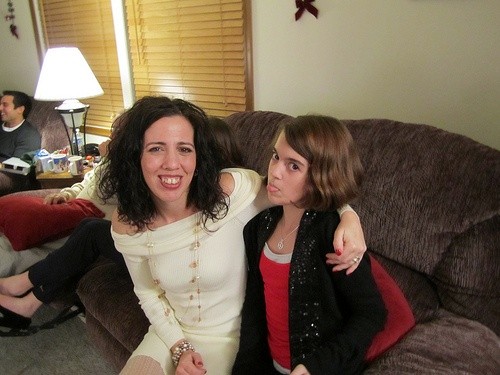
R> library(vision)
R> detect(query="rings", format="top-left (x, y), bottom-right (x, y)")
top-left (353, 255), bottom-right (359, 263)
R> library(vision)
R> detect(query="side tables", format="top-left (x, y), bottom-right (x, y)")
top-left (36, 156), bottom-right (94, 190)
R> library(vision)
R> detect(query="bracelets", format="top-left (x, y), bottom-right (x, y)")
top-left (171, 341), bottom-right (195, 367)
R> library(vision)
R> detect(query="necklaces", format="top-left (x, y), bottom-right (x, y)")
top-left (147, 208), bottom-right (202, 328)
top-left (142, 210), bottom-right (195, 326)
top-left (277, 225), bottom-right (299, 250)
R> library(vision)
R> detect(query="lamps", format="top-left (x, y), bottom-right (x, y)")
top-left (34, 46), bottom-right (104, 166)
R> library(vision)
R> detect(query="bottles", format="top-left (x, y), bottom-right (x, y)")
top-left (73, 128), bottom-right (83, 152)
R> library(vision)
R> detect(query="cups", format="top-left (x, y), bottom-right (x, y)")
top-left (67, 156), bottom-right (83, 176)
top-left (47, 154), bottom-right (67, 173)
top-left (37, 156), bottom-right (51, 173)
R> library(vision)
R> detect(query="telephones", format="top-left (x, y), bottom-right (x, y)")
top-left (84, 143), bottom-right (99, 155)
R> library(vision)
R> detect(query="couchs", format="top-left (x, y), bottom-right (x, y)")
top-left (26, 100), bottom-right (72, 157)
top-left (52, 111), bottom-right (500, 375)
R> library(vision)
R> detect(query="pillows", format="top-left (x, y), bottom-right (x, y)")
top-left (0, 193), bottom-right (107, 251)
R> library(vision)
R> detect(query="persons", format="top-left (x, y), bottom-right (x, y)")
top-left (99, 97), bottom-right (368, 375)
top-left (229, 114), bottom-right (388, 375)
top-left (0, 89), bottom-right (245, 326)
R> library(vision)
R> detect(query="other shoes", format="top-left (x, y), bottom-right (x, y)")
top-left (0, 305), bottom-right (31, 329)
